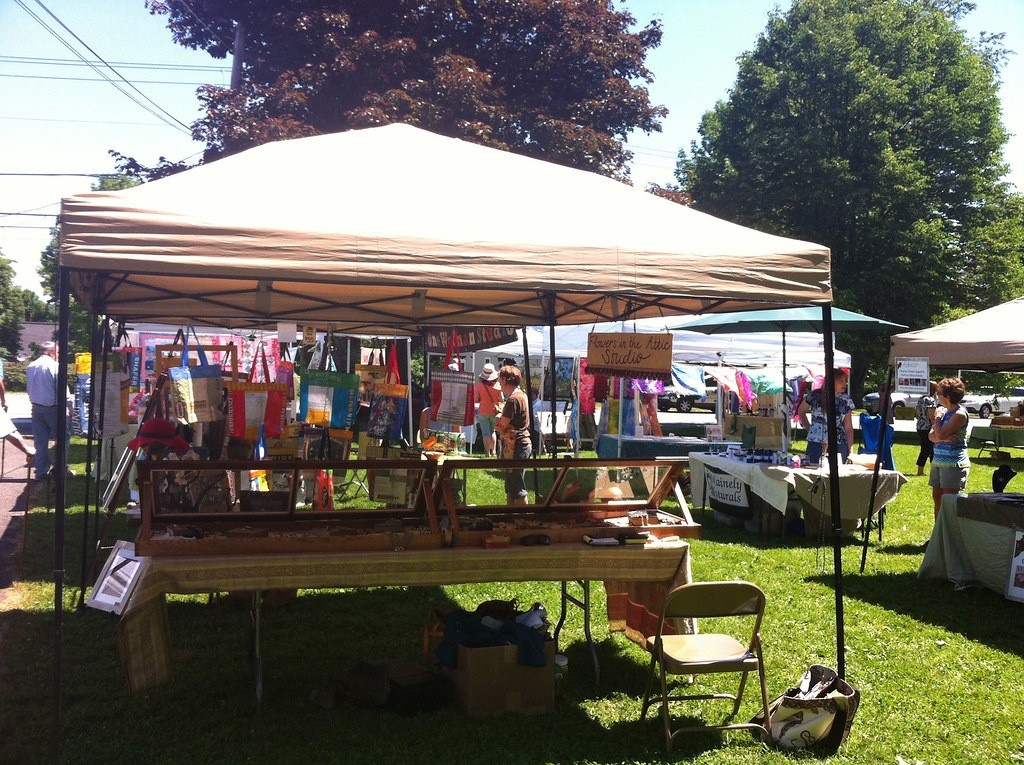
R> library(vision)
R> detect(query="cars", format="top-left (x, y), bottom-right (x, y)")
top-left (861, 385), bottom-right (941, 417)
top-left (657, 373), bottom-right (744, 415)
top-left (958, 384), bottom-right (1024, 419)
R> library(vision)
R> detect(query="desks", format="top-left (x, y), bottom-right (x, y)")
top-left (970, 425), bottom-right (1024, 461)
top-left (89, 425), bottom-right (142, 482)
top-left (594, 434), bottom-right (745, 499)
top-left (917, 492), bottom-right (1024, 603)
top-left (687, 452), bottom-right (908, 540)
top-left (125, 505), bottom-right (700, 709)
top-left (719, 415), bottom-right (792, 452)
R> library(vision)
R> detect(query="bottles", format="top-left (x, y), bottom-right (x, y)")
top-left (554, 654), bottom-right (569, 688)
top-left (725, 446), bottom-right (801, 468)
top-left (253, 422), bottom-right (267, 460)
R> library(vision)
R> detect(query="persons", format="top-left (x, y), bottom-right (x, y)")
top-left (0, 358), bottom-right (38, 468)
top-left (929, 375), bottom-right (970, 526)
top-left (798, 368), bottom-right (853, 464)
top-left (473, 363), bottom-right (541, 514)
top-left (914, 379), bottom-right (938, 477)
top-left (26, 340), bottom-right (77, 479)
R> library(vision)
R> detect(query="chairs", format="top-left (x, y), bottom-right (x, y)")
top-left (856, 413), bottom-right (895, 541)
top-left (638, 579), bottom-right (774, 754)
top-left (336, 429), bottom-right (382, 501)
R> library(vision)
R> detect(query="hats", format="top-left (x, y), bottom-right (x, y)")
top-left (127, 418), bottom-right (190, 454)
top-left (478, 363), bottom-right (499, 381)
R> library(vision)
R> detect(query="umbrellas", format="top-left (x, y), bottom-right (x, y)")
top-left (661, 305), bottom-right (909, 407)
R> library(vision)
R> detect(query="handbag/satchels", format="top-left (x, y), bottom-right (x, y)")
top-left (141, 323), bottom-right (476, 513)
top-left (748, 665), bottom-right (861, 753)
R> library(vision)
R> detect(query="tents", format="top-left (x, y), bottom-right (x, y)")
top-left (52, 121), bottom-right (851, 765)
top-left (859, 292), bottom-right (1024, 574)
top-left (540, 313), bottom-right (835, 464)
top-left (834, 349), bottom-right (851, 397)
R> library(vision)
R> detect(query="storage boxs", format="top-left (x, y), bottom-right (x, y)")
top-left (990, 451), bottom-right (1010, 461)
top-left (434, 626), bottom-right (555, 718)
top-left (707, 425), bottom-right (726, 443)
top-left (991, 407), bottom-right (1024, 428)
